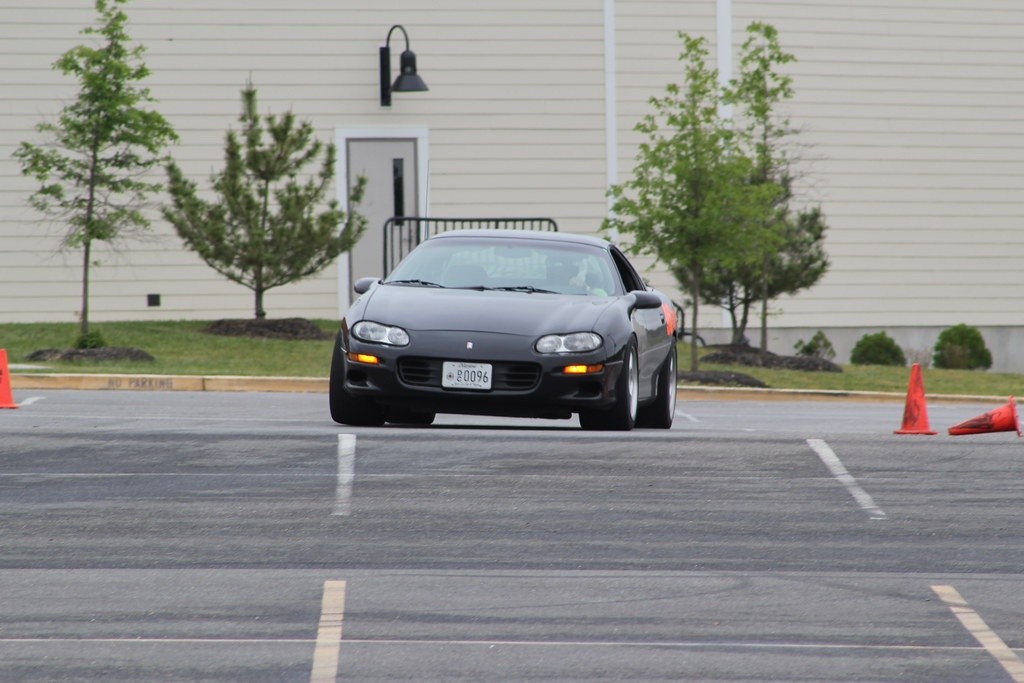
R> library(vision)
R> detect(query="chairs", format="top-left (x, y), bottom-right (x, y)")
top-left (444, 265), bottom-right (489, 286)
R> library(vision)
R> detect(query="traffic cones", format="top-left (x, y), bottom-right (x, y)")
top-left (893, 364), bottom-right (939, 436)
top-left (948, 395), bottom-right (1022, 438)
top-left (0, 347), bottom-right (20, 409)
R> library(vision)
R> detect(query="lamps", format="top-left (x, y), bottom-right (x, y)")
top-left (378, 25), bottom-right (428, 107)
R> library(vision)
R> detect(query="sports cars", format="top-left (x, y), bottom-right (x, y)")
top-left (327, 225), bottom-right (682, 432)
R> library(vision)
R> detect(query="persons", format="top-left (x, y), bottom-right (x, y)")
top-left (547, 255), bottom-right (607, 297)
